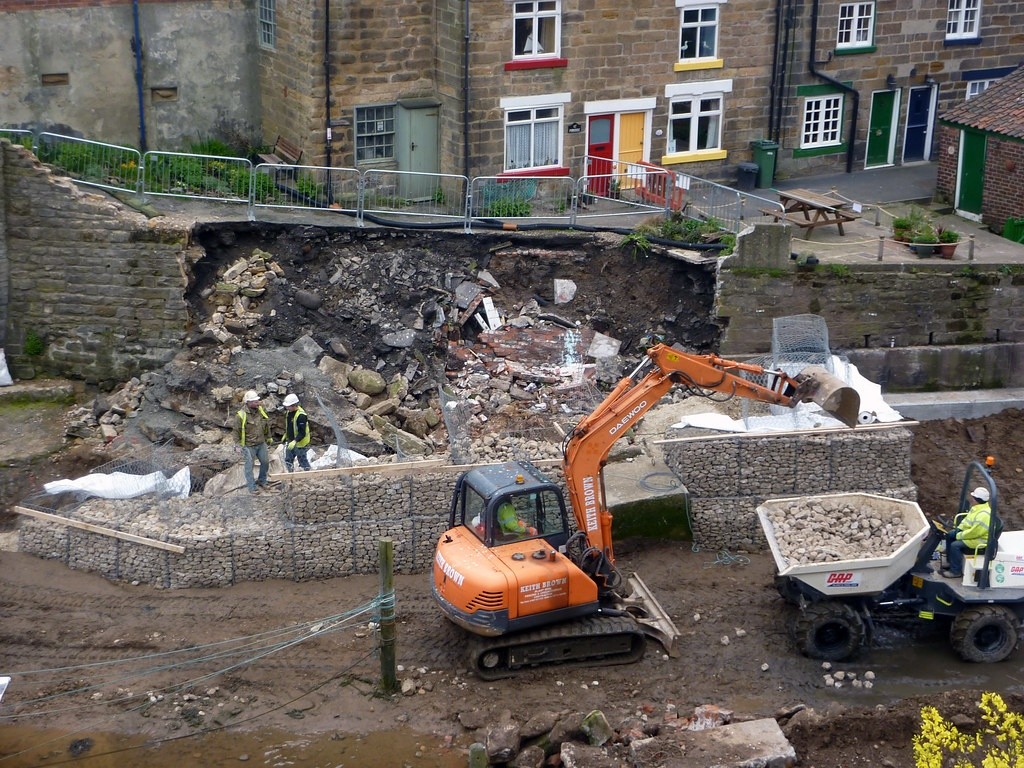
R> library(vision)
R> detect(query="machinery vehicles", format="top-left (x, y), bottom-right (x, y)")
top-left (754, 459), bottom-right (1024, 668)
top-left (426, 340), bottom-right (862, 683)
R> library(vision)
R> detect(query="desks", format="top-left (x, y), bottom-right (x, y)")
top-left (776, 189), bottom-right (850, 241)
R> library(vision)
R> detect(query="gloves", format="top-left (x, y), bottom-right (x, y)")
top-left (287, 440), bottom-right (297, 450)
top-left (267, 437), bottom-right (273, 445)
top-left (947, 528), bottom-right (957, 538)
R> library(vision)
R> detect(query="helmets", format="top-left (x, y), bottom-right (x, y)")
top-left (970, 486), bottom-right (990, 502)
top-left (242, 390), bottom-right (261, 402)
top-left (282, 393), bottom-right (301, 407)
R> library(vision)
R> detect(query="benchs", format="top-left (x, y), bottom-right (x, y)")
top-left (838, 210), bottom-right (862, 221)
top-left (256, 135), bottom-right (304, 185)
top-left (758, 207), bottom-right (815, 228)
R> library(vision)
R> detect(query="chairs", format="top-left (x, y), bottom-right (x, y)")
top-left (495, 520), bottom-right (519, 543)
top-left (962, 516), bottom-right (1005, 556)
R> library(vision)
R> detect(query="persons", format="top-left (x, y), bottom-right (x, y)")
top-left (493, 492), bottom-right (537, 537)
top-left (281, 394), bottom-right (313, 473)
top-left (231, 390), bottom-right (274, 497)
top-left (941, 486), bottom-right (995, 577)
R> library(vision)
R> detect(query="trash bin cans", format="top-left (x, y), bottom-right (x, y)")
top-left (748, 139), bottom-right (780, 189)
top-left (735, 162), bottom-right (759, 192)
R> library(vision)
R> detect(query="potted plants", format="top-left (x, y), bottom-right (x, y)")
top-left (608, 179), bottom-right (621, 199)
top-left (889, 207), bottom-right (961, 261)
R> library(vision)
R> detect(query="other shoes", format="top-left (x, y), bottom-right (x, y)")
top-left (940, 563), bottom-right (951, 570)
top-left (942, 570), bottom-right (962, 578)
top-left (260, 482), bottom-right (271, 490)
top-left (249, 489), bottom-right (260, 495)
